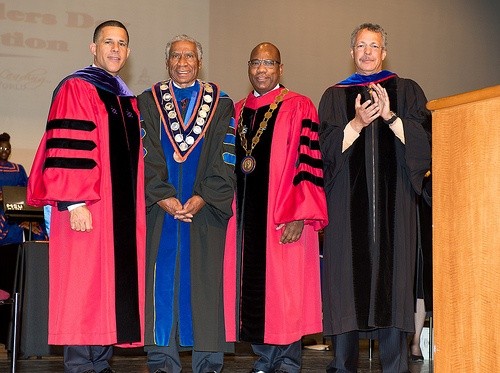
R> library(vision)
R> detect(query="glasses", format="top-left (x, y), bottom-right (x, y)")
top-left (248, 58), bottom-right (280, 67)
top-left (0, 146), bottom-right (10, 151)
top-left (355, 43), bottom-right (384, 52)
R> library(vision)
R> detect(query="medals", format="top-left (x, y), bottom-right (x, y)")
top-left (241, 154), bottom-right (256, 174)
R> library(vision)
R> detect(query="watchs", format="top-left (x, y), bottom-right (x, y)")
top-left (383, 111), bottom-right (397, 125)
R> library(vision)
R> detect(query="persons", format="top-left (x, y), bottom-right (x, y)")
top-left (318, 22), bottom-right (432, 373)
top-left (137, 34), bottom-right (237, 373)
top-left (0, 133), bottom-right (49, 245)
top-left (27, 19), bottom-right (145, 373)
top-left (224, 42), bottom-right (329, 373)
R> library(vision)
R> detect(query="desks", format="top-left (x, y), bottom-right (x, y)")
top-left (0, 243), bottom-right (64, 360)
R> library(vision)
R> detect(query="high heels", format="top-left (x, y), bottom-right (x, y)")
top-left (409, 341), bottom-right (424, 362)
